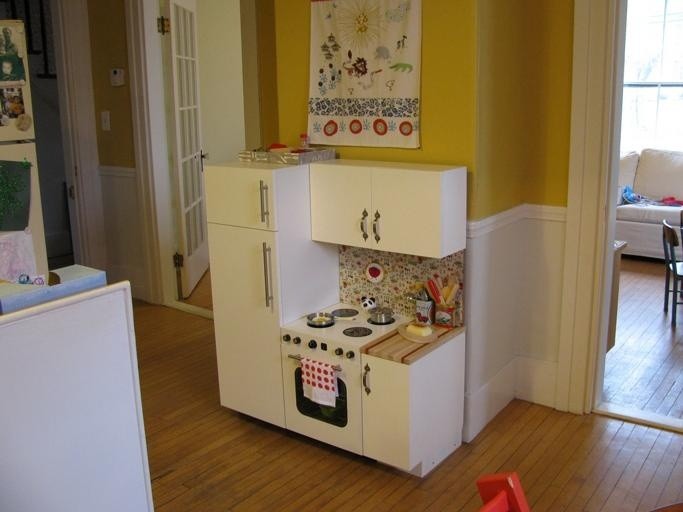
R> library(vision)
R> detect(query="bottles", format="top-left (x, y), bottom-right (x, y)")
top-left (300, 133), bottom-right (307, 148)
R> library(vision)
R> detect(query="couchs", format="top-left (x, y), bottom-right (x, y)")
top-left (616, 147), bottom-right (683, 263)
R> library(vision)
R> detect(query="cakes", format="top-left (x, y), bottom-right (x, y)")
top-left (405, 322), bottom-right (432, 337)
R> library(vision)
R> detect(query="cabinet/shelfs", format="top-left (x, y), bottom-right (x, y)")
top-left (202, 159), bottom-right (468, 480)
top-left (607, 239), bottom-right (627, 354)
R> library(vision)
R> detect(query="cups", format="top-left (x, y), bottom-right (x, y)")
top-left (436, 304), bottom-right (457, 328)
top-left (415, 300), bottom-right (434, 328)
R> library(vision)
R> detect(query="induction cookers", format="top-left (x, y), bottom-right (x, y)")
top-left (281, 303), bottom-right (406, 365)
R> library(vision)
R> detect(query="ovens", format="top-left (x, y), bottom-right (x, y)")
top-left (280, 348), bottom-right (361, 458)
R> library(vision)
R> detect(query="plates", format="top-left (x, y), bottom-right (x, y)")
top-left (306, 312), bottom-right (333, 326)
top-left (398, 322), bottom-right (437, 345)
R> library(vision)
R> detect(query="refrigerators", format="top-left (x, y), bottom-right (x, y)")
top-left (202, 150), bottom-right (339, 431)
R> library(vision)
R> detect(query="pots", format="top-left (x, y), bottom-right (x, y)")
top-left (368, 306), bottom-right (395, 324)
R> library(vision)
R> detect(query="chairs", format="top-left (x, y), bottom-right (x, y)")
top-left (662, 210), bottom-right (683, 327)
